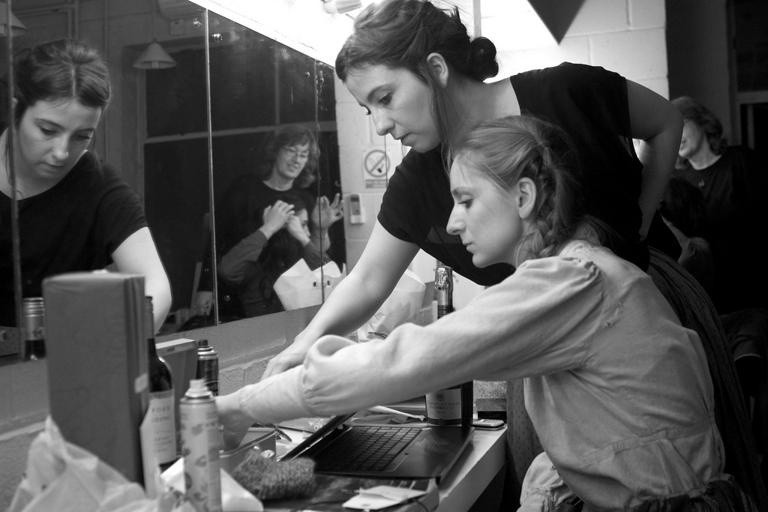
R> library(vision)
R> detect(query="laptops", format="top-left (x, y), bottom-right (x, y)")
top-left (278, 412), bottom-right (475, 485)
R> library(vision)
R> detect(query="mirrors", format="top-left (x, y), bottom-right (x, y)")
top-left (1, 0), bottom-right (351, 366)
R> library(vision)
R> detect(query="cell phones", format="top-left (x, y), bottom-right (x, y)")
top-left (472, 418), bottom-right (505, 431)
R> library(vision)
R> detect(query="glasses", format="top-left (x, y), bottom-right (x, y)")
top-left (281, 145), bottom-right (308, 161)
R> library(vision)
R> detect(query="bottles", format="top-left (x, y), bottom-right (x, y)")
top-left (21, 295), bottom-right (45, 362)
top-left (422, 264), bottom-right (476, 436)
top-left (197, 337), bottom-right (220, 398)
top-left (179, 375), bottom-right (225, 512)
top-left (140, 293), bottom-right (177, 473)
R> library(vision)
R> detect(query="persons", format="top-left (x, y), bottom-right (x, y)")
top-left (0, 40), bottom-right (172, 335)
